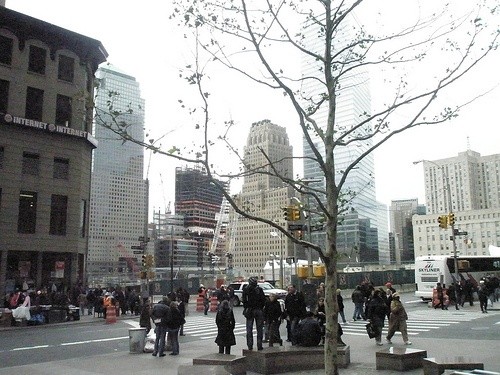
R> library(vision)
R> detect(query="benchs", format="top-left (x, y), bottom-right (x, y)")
top-left (243, 345), bottom-right (351, 375)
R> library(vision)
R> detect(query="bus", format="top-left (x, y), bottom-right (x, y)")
top-left (414, 255), bottom-right (500, 302)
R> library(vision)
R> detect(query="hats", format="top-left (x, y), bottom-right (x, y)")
top-left (392, 293), bottom-right (399, 298)
top-left (248, 276), bottom-right (259, 284)
top-left (386, 283), bottom-right (391, 286)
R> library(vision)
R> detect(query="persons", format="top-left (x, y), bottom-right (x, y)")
top-left (0, 277), bottom-right (411, 356)
top-left (434, 275), bottom-right (500, 313)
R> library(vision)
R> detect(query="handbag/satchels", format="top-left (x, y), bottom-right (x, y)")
top-left (242, 308), bottom-right (252, 318)
top-left (366, 322), bottom-right (378, 338)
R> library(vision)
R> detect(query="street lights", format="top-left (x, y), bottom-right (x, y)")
top-left (411, 159), bottom-right (459, 281)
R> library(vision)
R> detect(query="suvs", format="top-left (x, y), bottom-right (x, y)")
top-left (226, 280), bottom-right (288, 307)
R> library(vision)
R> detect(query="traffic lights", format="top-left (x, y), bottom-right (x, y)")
top-left (142, 255), bottom-right (150, 267)
top-left (438, 216), bottom-right (447, 228)
top-left (292, 206), bottom-right (301, 221)
top-left (284, 206), bottom-right (295, 220)
top-left (449, 213), bottom-right (455, 225)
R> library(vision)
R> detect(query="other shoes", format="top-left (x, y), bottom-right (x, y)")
top-left (386, 338), bottom-right (392, 343)
top-left (376, 342), bottom-right (383, 345)
top-left (152, 353), bottom-right (157, 356)
top-left (357, 316), bottom-right (361, 319)
top-left (159, 354), bottom-right (166, 357)
top-left (170, 351), bottom-right (179, 355)
top-left (404, 340), bottom-right (412, 345)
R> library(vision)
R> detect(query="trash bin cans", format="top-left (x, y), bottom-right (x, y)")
top-left (129, 328), bottom-right (146, 353)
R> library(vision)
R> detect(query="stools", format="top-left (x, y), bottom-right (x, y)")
top-left (423, 357), bottom-right (484, 375)
top-left (193, 353), bottom-right (247, 375)
top-left (178, 364), bottom-right (231, 375)
top-left (376, 347), bottom-right (427, 371)
